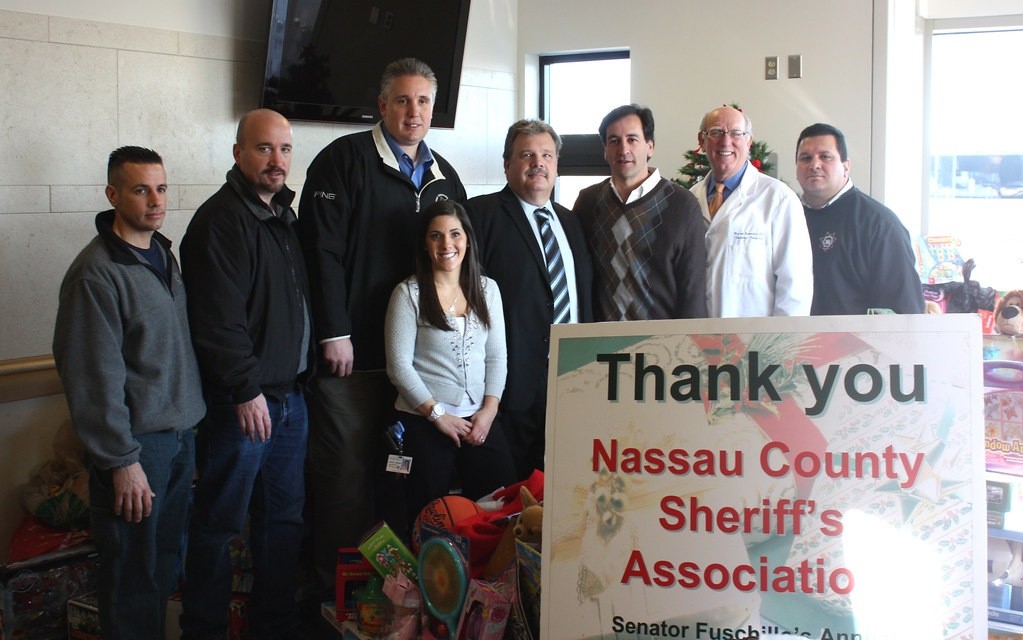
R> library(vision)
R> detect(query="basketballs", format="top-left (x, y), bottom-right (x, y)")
top-left (410, 495), bottom-right (486, 555)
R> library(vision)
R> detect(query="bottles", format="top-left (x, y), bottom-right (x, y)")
top-left (357, 573), bottom-right (392, 635)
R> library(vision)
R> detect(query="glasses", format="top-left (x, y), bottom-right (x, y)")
top-left (702, 129), bottom-right (748, 139)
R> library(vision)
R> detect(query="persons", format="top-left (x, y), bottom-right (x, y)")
top-left (52, 145), bottom-right (208, 640)
top-left (385, 201), bottom-right (508, 510)
top-left (467, 118), bottom-right (595, 481)
top-left (571, 105), bottom-right (708, 322)
top-left (688, 106), bottom-right (815, 318)
top-left (179, 108), bottom-right (317, 640)
top-left (796, 123), bottom-right (927, 317)
top-left (298, 58), bottom-right (467, 640)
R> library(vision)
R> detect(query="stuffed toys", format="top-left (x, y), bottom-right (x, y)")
top-left (512, 486), bottom-right (543, 543)
top-left (992, 289), bottom-right (1023, 336)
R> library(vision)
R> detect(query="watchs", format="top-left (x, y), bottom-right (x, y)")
top-left (426, 403), bottom-right (446, 421)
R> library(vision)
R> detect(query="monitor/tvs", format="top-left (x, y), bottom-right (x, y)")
top-left (261, 0), bottom-right (471, 130)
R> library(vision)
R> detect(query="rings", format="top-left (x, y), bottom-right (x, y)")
top-left (480, 437), bottom-right (484, 443)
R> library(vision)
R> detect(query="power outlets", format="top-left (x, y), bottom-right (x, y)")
top-left (765, 56), bottom-right (778, 80)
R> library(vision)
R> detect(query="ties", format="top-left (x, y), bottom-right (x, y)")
top-left (534, 207), bottom-right (570, 325)
top-left (710, 184), bottom-right (724, 218)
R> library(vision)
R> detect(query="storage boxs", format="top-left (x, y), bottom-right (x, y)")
top-left (163, 592), bottom-right (250, 640)
top-left (357, 518), bottom-right (419, 587)
top-left (67, 590), bottom-right (103, 640)
top-left (454, 578), bottom-right (511, 640)
top-left (336, 547), bottom-right (376, 620)
